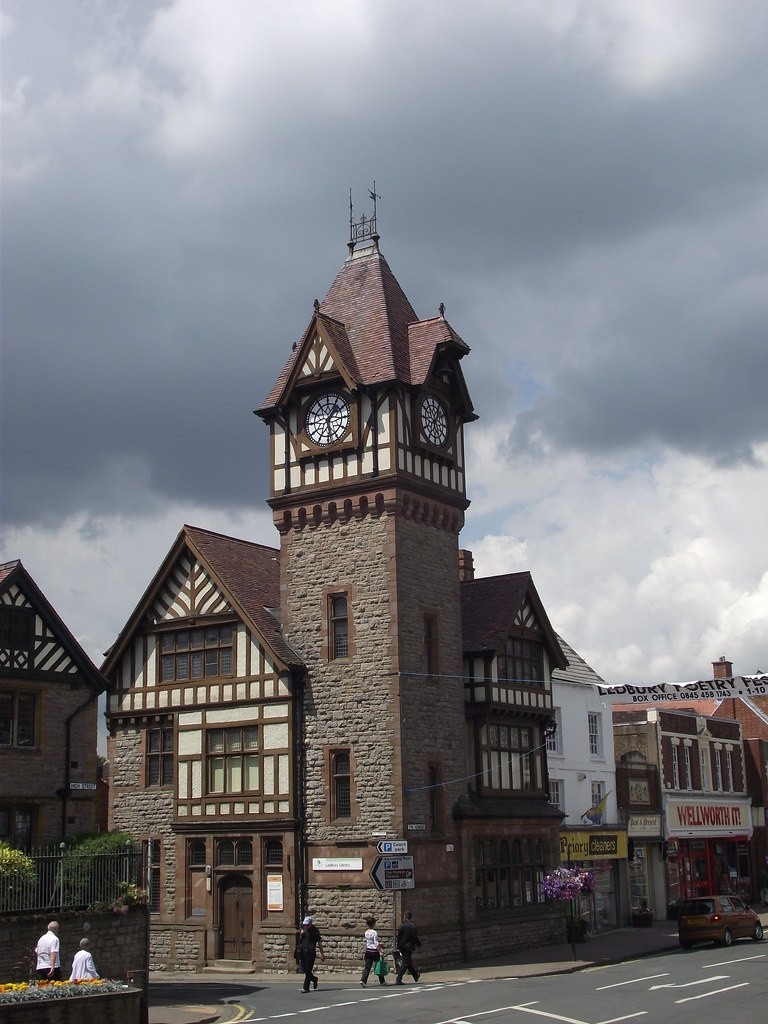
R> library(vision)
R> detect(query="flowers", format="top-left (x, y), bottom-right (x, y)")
top-left (630, 904), bottom-right (656, 914)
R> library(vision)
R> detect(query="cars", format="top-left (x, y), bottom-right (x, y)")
top-left (677, 895), bottom-right (763, 952)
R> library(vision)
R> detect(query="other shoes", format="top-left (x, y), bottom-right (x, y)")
top-left (415, 972), bottom-right (420, 981)
top-left (302, 990), bottom-right (310, 993)
top-left (360, 981), bottom-right (367, 988)
top-left (396, 981), bottom-right (405, 985)
top-left (380, 981), bottom-right (387, 985)
top-left (314, 977), bottom-right (318, 989)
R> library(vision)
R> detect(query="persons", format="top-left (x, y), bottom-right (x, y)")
top-left (35, 921), bottom-right (61, 980)
top-left (395, 910), bottom-right (421, 984)
top-left (69, 938), bottom-right (100, 980)
top-left (759, 870), bottom-right (768, 907)
top-left (359, 916), bottom-right (388, 987)
top-left (298, 917), bottom-right (325, 993)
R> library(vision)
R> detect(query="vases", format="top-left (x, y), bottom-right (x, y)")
top-left (631, 912), bottom-right (653, 927)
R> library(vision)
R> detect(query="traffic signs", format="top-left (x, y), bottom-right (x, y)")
top-left (369, 855), bottom-right (416, 891)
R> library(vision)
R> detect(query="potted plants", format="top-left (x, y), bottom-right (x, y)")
top-left (567, 918), bottom-right (588, 943)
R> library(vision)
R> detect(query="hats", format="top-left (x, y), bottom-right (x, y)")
top-left (303, 917), bottom-right (312, 924)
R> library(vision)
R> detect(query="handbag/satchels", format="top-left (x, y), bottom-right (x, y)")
top-left (374, 956), bottom-right (389, 975)
top-left (294, 944), bottom-right (303, 964)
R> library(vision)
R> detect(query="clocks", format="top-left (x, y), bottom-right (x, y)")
top-left (411, 384), bottom-right (458, 464)
top-left (296, 380), bottom-right (361, 460)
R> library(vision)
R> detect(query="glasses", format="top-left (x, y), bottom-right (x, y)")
top-left (304, 923), bottom-right (311, 926)
